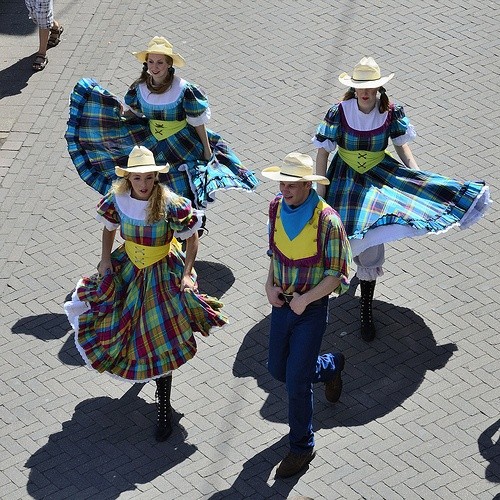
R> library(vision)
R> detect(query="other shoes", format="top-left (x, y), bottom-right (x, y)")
top-left (276, 448), bottom-right (316, 476)
top-left (324, 352), bottom-right (344, 402)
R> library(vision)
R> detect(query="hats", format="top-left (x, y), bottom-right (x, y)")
top-left (339, 56), bottom-right (395, 89)
top-left (136, 36), bottom-right (184, 67)
top-left (114, 144), bottom-right (169, 177)
top-left (261, 151), bottom-right (330, 187)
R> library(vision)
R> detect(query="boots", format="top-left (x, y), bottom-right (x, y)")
top-left (359, 278), bottom-right (377, 342)
top-left (152, 375), bottom-right (172, 441)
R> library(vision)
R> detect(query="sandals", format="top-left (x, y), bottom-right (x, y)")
top-left (32, 54), bottom-right (49, 71)
top-left (48, 26), bottom-right (64, 46)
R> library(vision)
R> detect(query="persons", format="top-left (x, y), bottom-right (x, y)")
top-left (312, 56), bottom-right (493, 342)
top-left (260, 152), bottom-right (353, 477)
top-left (25, 0), bottom-right (64, 71)
top-left (64, 36), bottom-right (258, 253)
top-left (63, 146), bottom-right (228, 442)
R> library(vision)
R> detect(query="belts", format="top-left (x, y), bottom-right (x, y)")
top-left (279, 294), bottom-right (294, 304)
